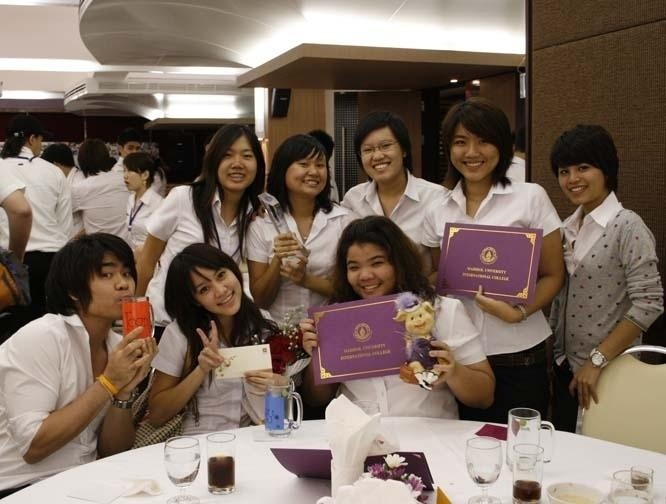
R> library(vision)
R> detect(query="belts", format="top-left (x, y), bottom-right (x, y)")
top-left (487, 348), bottom-right (546, 369)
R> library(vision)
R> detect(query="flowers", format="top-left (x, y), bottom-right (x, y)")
top-left (364, 450), bottom-right (424, 493)
top-left (259, 298), bottom-right (311, 376)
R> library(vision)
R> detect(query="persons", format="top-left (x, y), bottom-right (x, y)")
top-left (420, 96), bottom-right (565, 425)
top-left (300, 215), bottom-right (496, 419)
top-left (0, 232), bottom-right (160, 497)
top-left (341, 112), bottom-right (454, 303)
top-left (147, 242), bottom-right (295, 439)
top-left (0, 114), bottom-right (166, 348)
top-left (505, 128), bottom-right (528, 183)
top-left (136, 125), bottom-right (265, 345)
top-left (246, 134), bottom-right (355, 388)
top-left (307, 126), bottom-right (340, 203)
top-left (544, 124), bottom-right (665, 434)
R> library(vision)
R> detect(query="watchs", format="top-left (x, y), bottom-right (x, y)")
top-left (589, 347), bottom-right (609, 368)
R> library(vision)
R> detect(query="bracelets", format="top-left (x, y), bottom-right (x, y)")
top-left (96, 375), bottom-right (117, 402)
top-left (288, 378), bottom-right (294, 393)
top-left (113, 388), bottom-right (140, 408)
top-left (513, 303), bottom-right (528, 321)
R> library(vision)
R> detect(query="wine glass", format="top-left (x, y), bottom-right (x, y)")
top-left (161, 433), bottom-right (203, 504)
top-left (467, 436), bottom-right (504, 503)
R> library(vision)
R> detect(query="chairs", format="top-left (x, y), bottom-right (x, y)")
top-left (575, 343), bottom-right (665, 456)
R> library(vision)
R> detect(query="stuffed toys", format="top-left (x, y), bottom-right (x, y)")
top-left (393, 294), bottom-right (445, 390)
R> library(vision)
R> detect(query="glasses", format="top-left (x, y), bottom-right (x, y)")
top-left (356, 140), bottom-right (399, 158)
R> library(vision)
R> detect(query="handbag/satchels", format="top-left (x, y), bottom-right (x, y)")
top-left (130, 384), bottom-right (187, 451)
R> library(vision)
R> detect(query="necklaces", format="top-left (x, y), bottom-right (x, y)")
top-left (465, 193), bottom-right (484, 202)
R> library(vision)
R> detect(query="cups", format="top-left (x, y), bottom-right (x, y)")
top-left (632, 464), bottom-right (654, 495)
top-left (264, 381), bottom-right (306, 437)
top-left (351, 400), bottom-right (379, 415)
top-left (608, 470), bottom-right (653, 502)
top-left (207, 432), bottom-right (238, 494)
top-left (512, 444), bottom-right (546, 503)
top-left (120, 295), bottom-right (156, 343)
top-left (505, 407), bottom-right (554, 471)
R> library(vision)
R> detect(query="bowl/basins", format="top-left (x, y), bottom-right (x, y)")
top-left (547, 482), bottom-right (602, 503)
